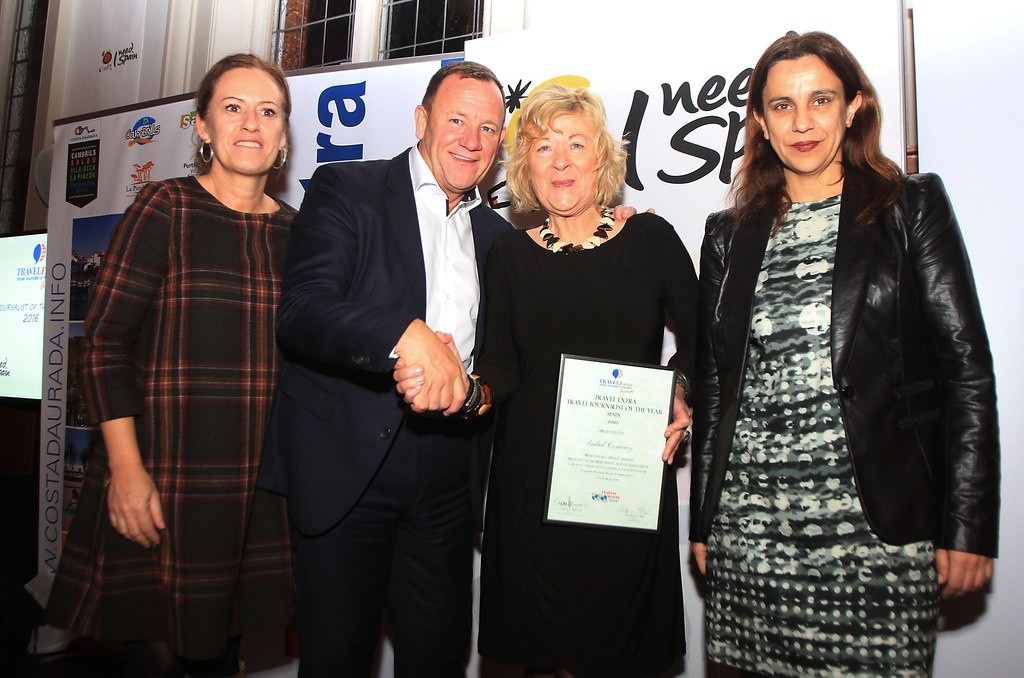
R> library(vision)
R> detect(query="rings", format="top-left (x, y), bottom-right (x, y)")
top-left (681, 429), bottom-right (691, 442)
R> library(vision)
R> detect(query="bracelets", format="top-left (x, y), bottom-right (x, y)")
top-left (462, 376), bottom-right (485, 416)
top-left (672, 374), bottom-right (692, 397)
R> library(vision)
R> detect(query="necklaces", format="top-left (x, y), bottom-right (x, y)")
top-left (538, 206), bottom-right (615, 255)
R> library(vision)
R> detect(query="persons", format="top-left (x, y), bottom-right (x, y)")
top-left (254, 63), bottom-right (655, 678)
top-left (392, 85), bottom-right (699, 678)
top-left (689, 31), bottom-right (1000, 678)
top-left (45, 53), bottom-right (299, 678)
